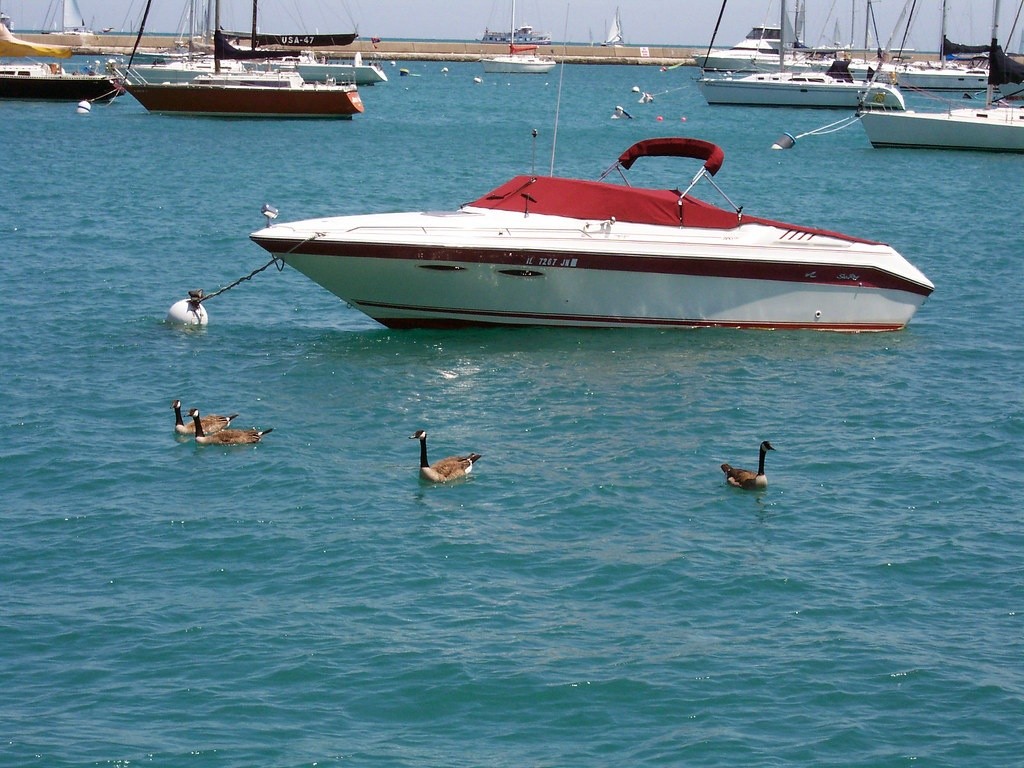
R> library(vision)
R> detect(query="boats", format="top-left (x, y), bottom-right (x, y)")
top-left (0, 59), bottom-right (124, 102)
top-left (471, 2), bottom-right (554, 44)
top-left (249, 138), bottom-right (936, 335)
top-left (111, 1), bottom-right (365, 117)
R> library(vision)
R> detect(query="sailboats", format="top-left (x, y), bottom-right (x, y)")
top-left (602, 4), bottom-right (626, 46)
top-left (110, 0), bottom-right (388, 83)
top-left (479, 0), bottom-right (557, 73)
top-left (691, 0), bottom-right (1024, 151)
top-left (41, 0), bottom-right (94, 36)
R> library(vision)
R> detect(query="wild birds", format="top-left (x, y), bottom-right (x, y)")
top-left (170, 400), bottom-right (274, 444)
top-left (408, 430), bottom-right (480, 483)
top-left (720, 441), bottom-right (775, 489)
